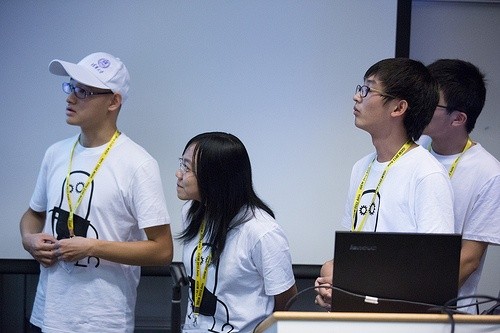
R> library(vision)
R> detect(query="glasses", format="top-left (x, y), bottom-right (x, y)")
top-left (355, 84), bottom-right (410, 111)
top-left (178, 157), bottom-right (196, 174)
top-left (62, 81), bottom-right (113, 99)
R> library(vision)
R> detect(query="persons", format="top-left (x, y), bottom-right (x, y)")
top-left (421, 57), bottom-right (500, 311)
top-left (20, 51), bottom-right (172, 333)
top-left (311, 57), bottom-right (456, 311)
top-left (172, 131), bottom-right (298, 333)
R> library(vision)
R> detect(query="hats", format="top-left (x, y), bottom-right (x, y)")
top-left (49, 52), bottom-right (130, 105)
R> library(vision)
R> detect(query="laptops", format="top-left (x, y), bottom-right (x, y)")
top-left (333, 230), bottom-right (463, 314)
